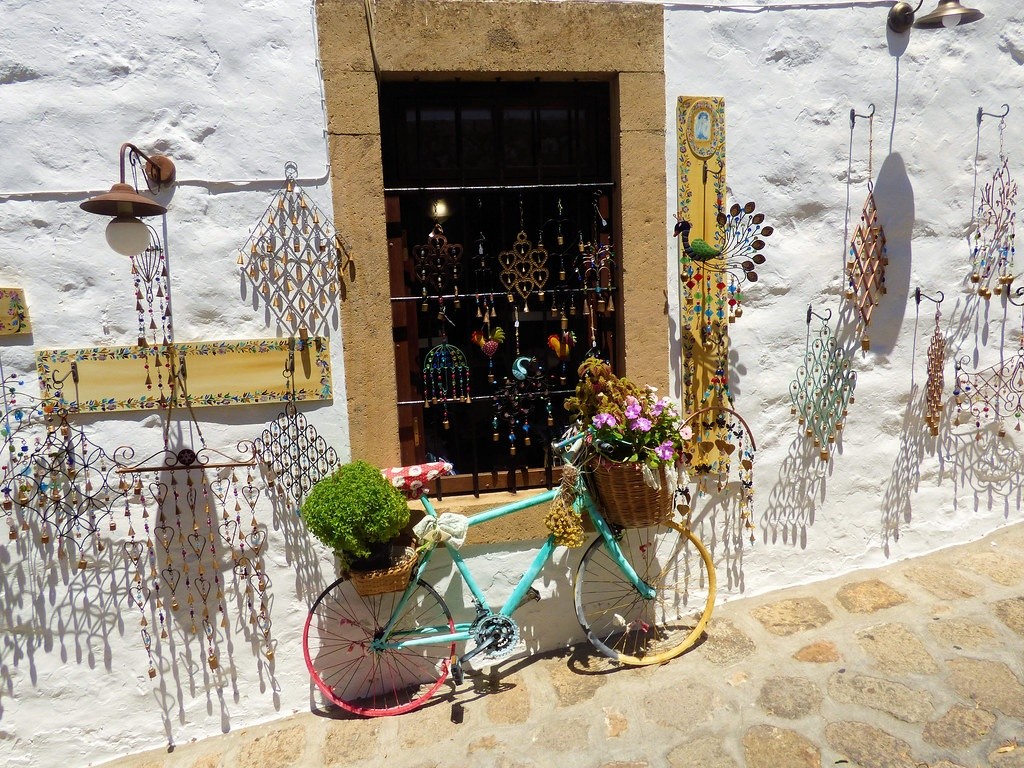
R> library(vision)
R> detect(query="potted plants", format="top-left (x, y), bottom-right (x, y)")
top-left (300, 460), bottom-right (410, 576)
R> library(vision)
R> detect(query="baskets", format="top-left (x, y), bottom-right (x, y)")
top-left (588, 455), bottom-right (674, 528)
top-left (343, 533), bottom-right (416, 596)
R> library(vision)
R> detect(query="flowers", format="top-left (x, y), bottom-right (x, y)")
top-left (544, 485), bottom-right (588, 547)
top-left (564, 354), bottom-right (694, 468)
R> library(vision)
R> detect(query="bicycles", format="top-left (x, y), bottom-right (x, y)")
top-left (302, 430), bottom-right (717, 717)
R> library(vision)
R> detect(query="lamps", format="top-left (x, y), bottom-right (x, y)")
top-left (887, 0), bottom-right (984, 34)
top-left (78, 144), bottom-right (176, 258)
